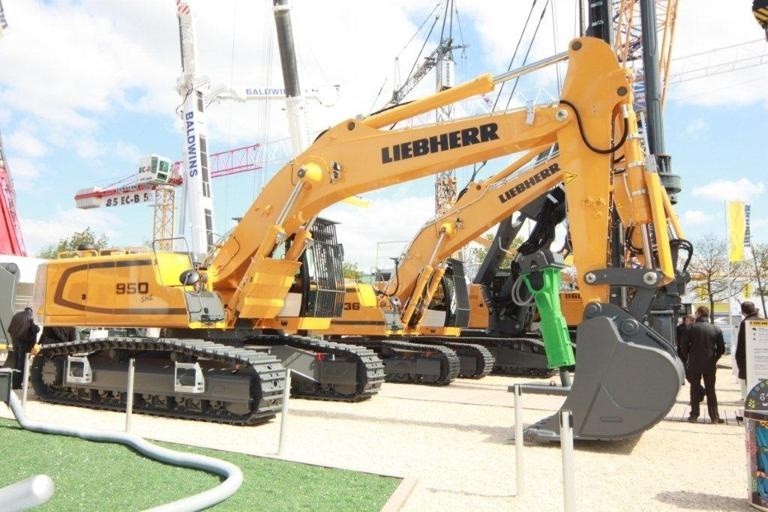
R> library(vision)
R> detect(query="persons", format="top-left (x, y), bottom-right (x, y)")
top-left (678, 305), bottom-right (726, 423)
top-left (734, 301), bottom-right (766, 387)
top-left (36, 322), bottom-right (74, 344)
top-left (8, 307), bottom-right (40, 389)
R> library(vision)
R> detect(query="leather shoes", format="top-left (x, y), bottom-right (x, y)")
top-left (687, 415), bottom-right (698, 422)
top-left (711, 417), bottom-right (725, 424)
